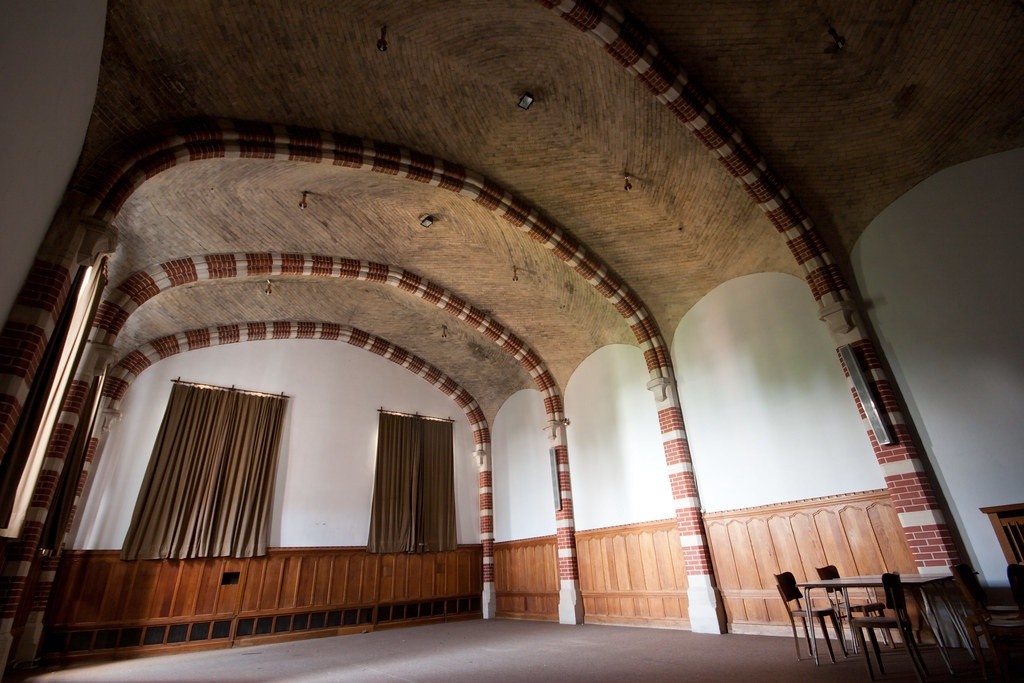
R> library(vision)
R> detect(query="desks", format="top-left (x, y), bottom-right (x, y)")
top-left (793, 575), bottom-right (977, 675)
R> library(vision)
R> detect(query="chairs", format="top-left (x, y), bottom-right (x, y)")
top-left (773, 571), bottom-right (848, 663)
top-left (849, 570), bottom-right (931, 683)
top-left (815, 565), bottom-right (896, 654)
top-left (962, 564), bottom-right (1024, 680)
top-left (948, 561), bottom-right (1024, 683)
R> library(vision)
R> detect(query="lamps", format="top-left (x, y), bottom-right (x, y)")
top-left (419, 216), bottom-right (433, 230)
top-left (517, 93), bottom-right (535, 111)
